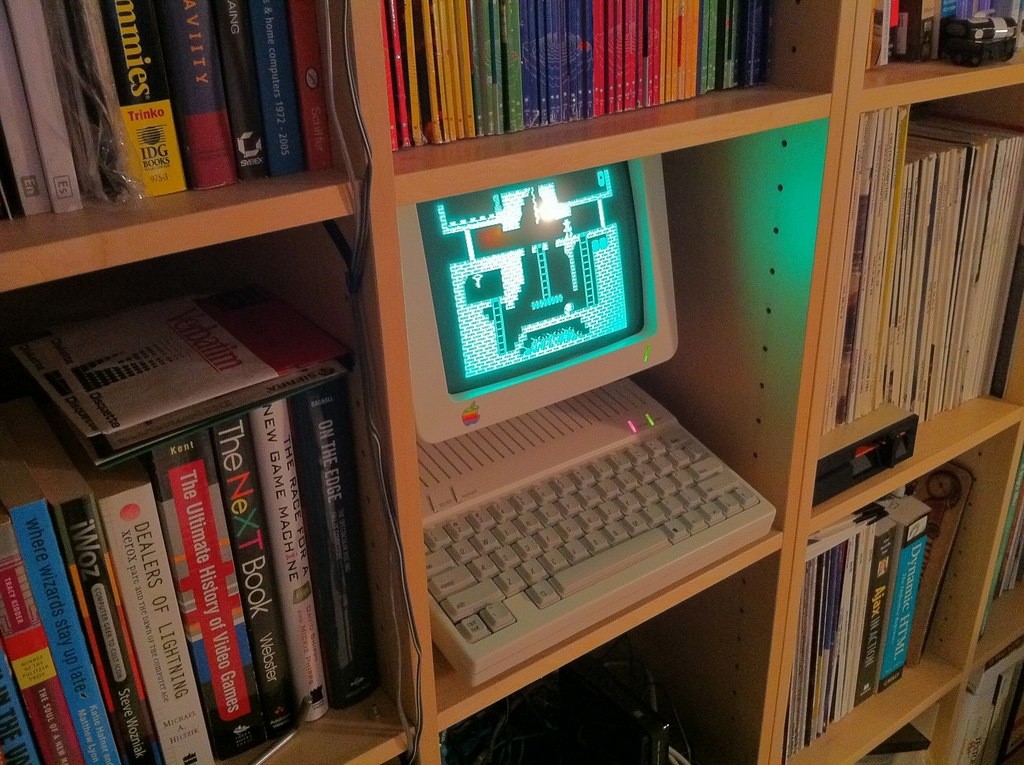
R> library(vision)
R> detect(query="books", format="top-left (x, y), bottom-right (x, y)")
top-left (865, 1), bottom-right (1024, 82)
top-left (0, 0), bottom-right (331, 223)
top-left (783, 464), bottom-right (974, 764)
top-left (1, 271), bottom-right (378, 765)
top-left (819, 101), bottom-right (1023, 433)
top-left (382, 1), bottom-right (777, 154)
top-left (945, 444), bottom-right (1024, 765)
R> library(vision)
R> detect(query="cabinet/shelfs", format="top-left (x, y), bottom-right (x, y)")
top-left (0, 2), bottom-right (1023, 765)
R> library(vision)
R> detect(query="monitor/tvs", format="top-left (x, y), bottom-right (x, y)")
top-left (397, 154), bottom-right (679, 447)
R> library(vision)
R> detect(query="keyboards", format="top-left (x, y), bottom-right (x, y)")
top-left (423, 429), bottom-right (775, 686)
